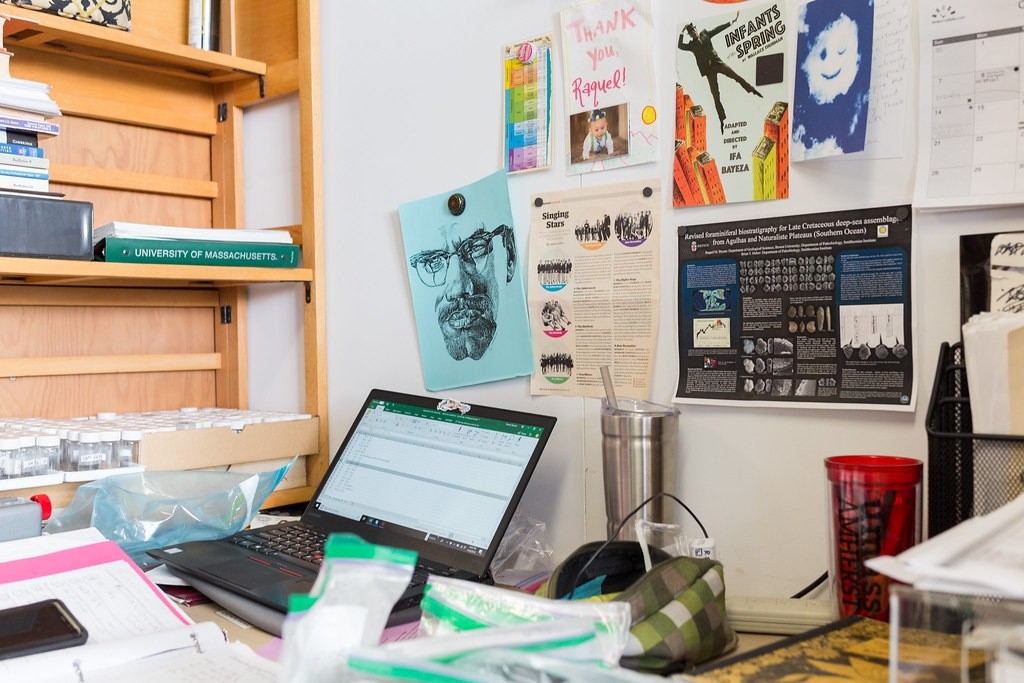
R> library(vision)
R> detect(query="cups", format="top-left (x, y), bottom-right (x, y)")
top-left (600, 397), bottom-right (682, 549)
top-left (824, 455), bottom-right (924, 624)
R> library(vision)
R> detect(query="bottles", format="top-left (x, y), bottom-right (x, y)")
top-left (0, 407), bottom-right (312, 481)
top-left (0, 494), bottom-right (52, 543)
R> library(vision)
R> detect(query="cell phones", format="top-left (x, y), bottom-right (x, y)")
top-left (0, 598), bottom-right (88, 661)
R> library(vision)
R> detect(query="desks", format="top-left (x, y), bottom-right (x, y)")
top-left (0, 528), bottom-right (991, 683)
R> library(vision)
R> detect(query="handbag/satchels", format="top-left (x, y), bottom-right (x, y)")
top-left (532, 540), bottom-right (738, 675)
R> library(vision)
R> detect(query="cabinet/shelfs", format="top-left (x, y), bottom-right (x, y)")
top-left (0, 0), bottom-right (330, 522)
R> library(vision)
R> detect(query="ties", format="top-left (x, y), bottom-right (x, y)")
top-left (596, 139), bottom-right (602, 152)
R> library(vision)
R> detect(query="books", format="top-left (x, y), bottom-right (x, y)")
top-left (94, 221), bottom-right (301, 269)
top-left (1, 14), bottom-right (65, 202)
top-left (0, 526), bottom-right (281, 683)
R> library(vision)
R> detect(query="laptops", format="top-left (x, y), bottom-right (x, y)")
top-left (145, 389), bottom-right (558, 616)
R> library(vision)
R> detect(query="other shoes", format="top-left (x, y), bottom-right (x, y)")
top-left (753, 90), bottom-right (763, 98)
top-left (721, 121), bottom-right (723, 135)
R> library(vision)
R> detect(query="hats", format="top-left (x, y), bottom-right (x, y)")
top-left (686, 23), bottom-right (696, 34)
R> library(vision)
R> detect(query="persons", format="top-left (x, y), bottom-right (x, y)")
top-left (615, 211), bottom-right (652, 240)
top-left (541, 300), bottom-right (571, 330)
top-left (678, 10), bottom-right (764, 135)
top-left (540, 353), bottom-right (574, 376)
top-left (582, 110), bottom-right (614, 159)
top-left (574, 214), bottom-right (612, 242)
top-left (538, 259), bottom-right (572, 284)
